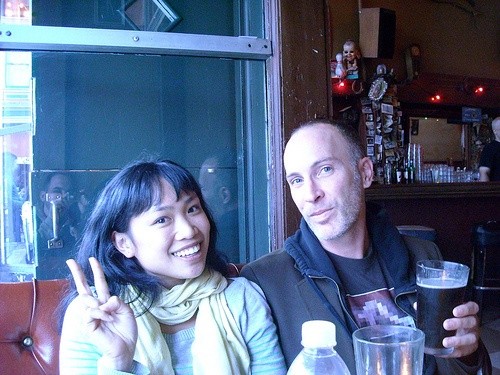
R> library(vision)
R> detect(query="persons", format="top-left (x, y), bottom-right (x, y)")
top-left (199, 155), bottom-right (237, 264)
top-left (238, 122), bottom-right (494, 375)
top-left (33, 171), bottom-right (91, 281)
top-left (478, 117), bottom-right (500, 181)
top-left (341, 39), bottom-right (360, 79)
top-left (57, 158), bottom-right (287, 375)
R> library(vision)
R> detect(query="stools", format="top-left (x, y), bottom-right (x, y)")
top-left (473, 220), bottom-right (500, 327)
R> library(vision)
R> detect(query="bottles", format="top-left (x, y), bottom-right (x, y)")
top-left (287, 321), bottom-right (351, 375)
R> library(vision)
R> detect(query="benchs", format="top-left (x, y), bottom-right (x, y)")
top-left (0, 262), bottom-right (246, 375)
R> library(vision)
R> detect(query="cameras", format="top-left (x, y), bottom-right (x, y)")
top-left (46, 193), bottom-right (62, 204)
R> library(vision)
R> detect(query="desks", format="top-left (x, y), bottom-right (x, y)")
top-left (365, 181), bottom-right (500, 200)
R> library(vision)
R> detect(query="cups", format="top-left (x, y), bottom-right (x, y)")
top-left (415, 260), bottom-right (470, 356)
top-left (384, 163), bottom-right (392, 184)
top-left (395, 170), bottom-right (401, 182)
top-left (353, 325), bottom-right (425, 375)
top-left (405, 142), bottom-right (480, 182)
